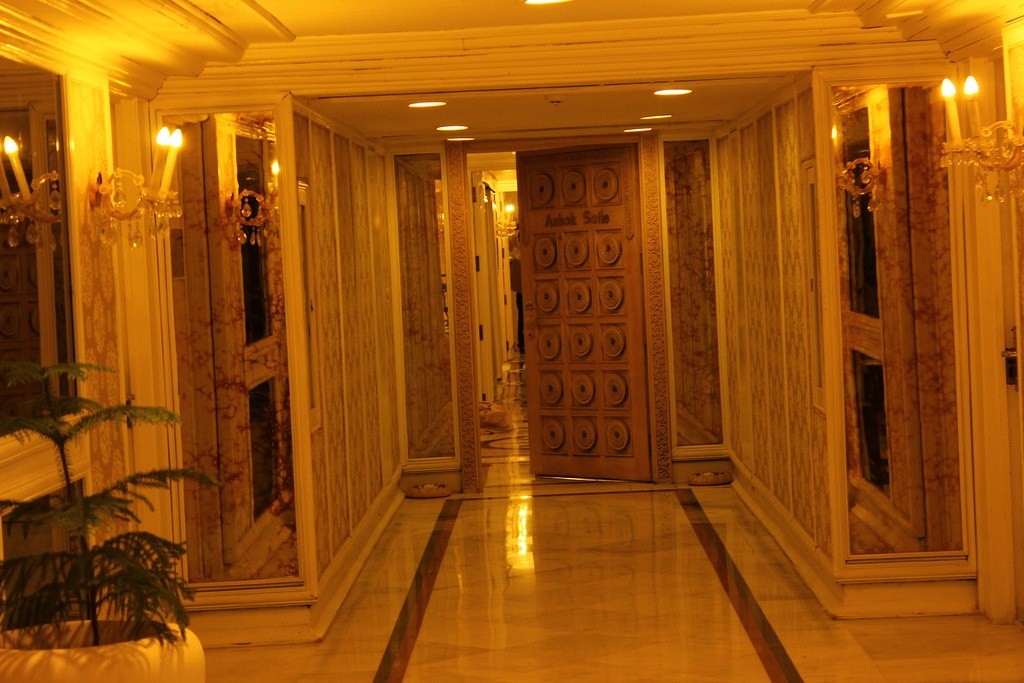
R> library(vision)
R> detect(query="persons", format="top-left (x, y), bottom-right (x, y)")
top-left (507, 221), bottom-right (525, 356)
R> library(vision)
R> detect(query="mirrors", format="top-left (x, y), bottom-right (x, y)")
top-left (663, 139), bottom-right (723, 447)
top-left (831, 79), bottom-right (964, 554)
top-left (393, 152), bottom-right (457, 460)
top-left (0, 478), bottom-right (92, 628)
top-left (234, 135), bottom-right (272, 347)
top-left (249, 379), bottom-right (280, 519)
top-left (0, 57), bottom-right (78, 435)
top-left (156, 102), bottom-right (299, 584)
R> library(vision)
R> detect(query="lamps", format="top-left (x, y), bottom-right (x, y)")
top-left (940, 76), bottom-right (1024, 210)
top-left (832, 124), bottom-right (886, 219)
top-left (496, 204), bottom-right (518, 240)
top-left (223, 159), bottom-right (279, 246)
top-left (90, 127), bottom-right (183, 251)
top-left (0, 135), bottom-right (63, 251)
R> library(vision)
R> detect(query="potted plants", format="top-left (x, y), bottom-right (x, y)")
top-left (0, 358), bottom-right (224, 683)
